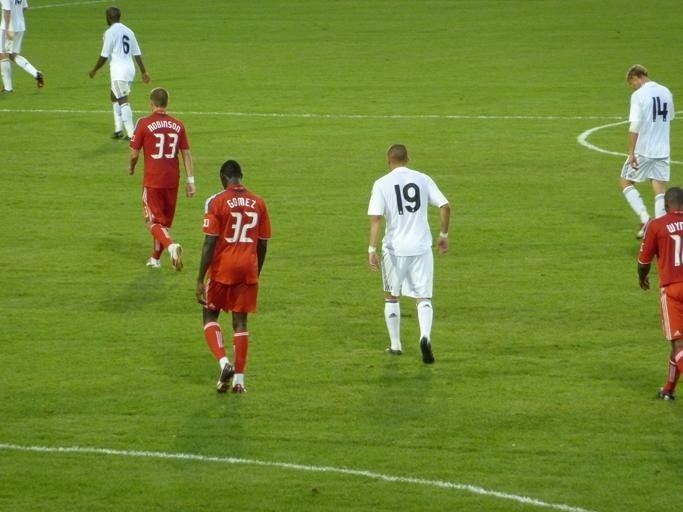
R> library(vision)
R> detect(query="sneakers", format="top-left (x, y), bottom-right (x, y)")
top-left (145, 256), bottom-right (160, 269)
top-left (216, 363), bottom-right (247, 393)
top-left (110, 130), bottom-right (131, 140)
top-left (636, 218), bottom-right (651, 239)
top-left (420, 336), bottom-right (434, 363)
top-left (170, 244), bottom-right (183, 271)
top-left (35, 72), bottom-right (45, 88)
top-left (385, 346), bottom-right (402, 355)
top-left (0, 87), bottom-right (12, 95)
top-left (658, 388), bottom-right (675, 400)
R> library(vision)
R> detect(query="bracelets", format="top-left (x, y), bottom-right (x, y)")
top-left (369, 246), bottom-right (376, 253)
top-left (440, 232), bottom-right (448, 238)
top-left (186, 176), bottom-right (194, 184)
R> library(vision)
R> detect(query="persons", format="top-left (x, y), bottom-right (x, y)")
top-left (620, 65), bottom-right (675, 238)
top-left (637, 187), bottom-right (683, 400)
top-left (129, 88), bottom-right (196, 271)
top-left (367, 145), bottom-right (450, 363)
top-left (196, 161), bottom-right (271, 393)
top-left (89, 8), bottom-right (149, 140)
top-left (0, 0), bottom-right (44, 93)
